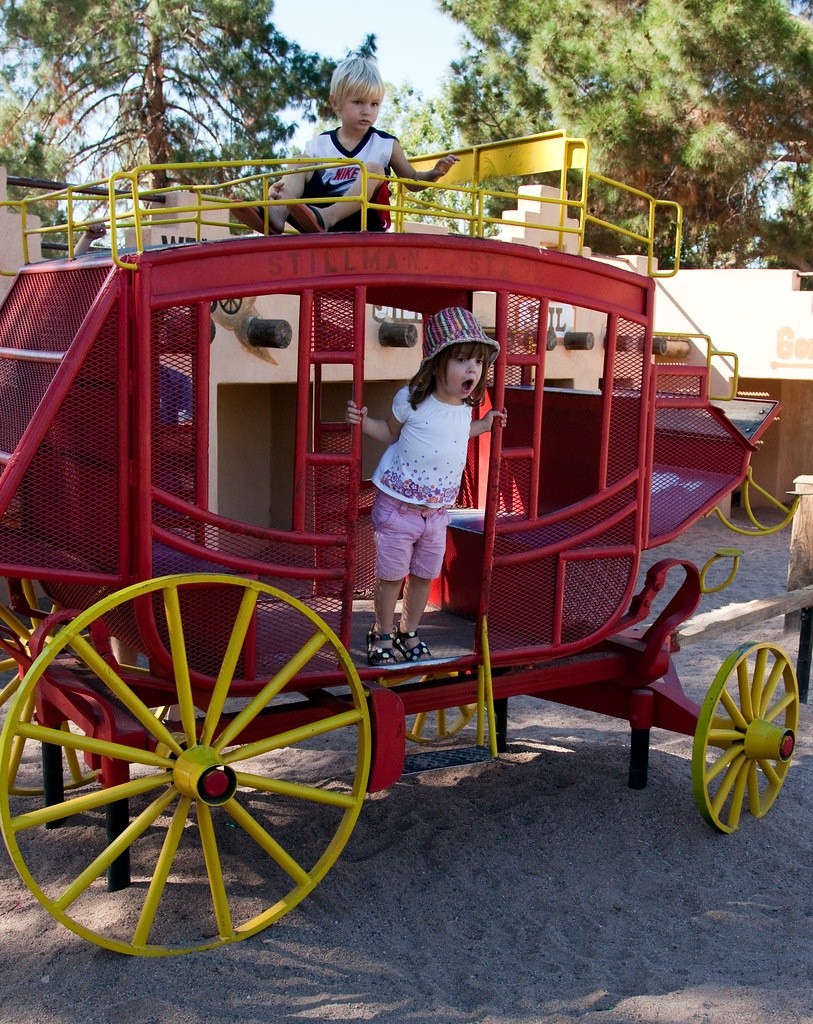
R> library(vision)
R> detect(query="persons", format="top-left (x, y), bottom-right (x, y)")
top-left (345, 306), bottom-right (507, 665)
top-left (71, 223), bottom-right (243, 580)
top-left (229, 58), bottom-right (460, 234)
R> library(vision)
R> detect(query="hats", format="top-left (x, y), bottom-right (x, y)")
top-left (419, 307), bottom-right (500, 369)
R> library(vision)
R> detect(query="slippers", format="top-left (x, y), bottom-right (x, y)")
top-left (286, 198), bottom-right (329, 234)
top-left (230, 198), bottom-right (284, 236)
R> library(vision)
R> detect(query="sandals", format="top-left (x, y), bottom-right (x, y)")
top-left (393, 621), bottom-right (434, 663)
top-left (365, 621), bottom-right (398, 666)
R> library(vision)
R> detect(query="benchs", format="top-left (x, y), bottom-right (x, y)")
top-left (403, 506), bottom-right (590, 629)
top-left (152, 541), bottom-right (257, 680)
